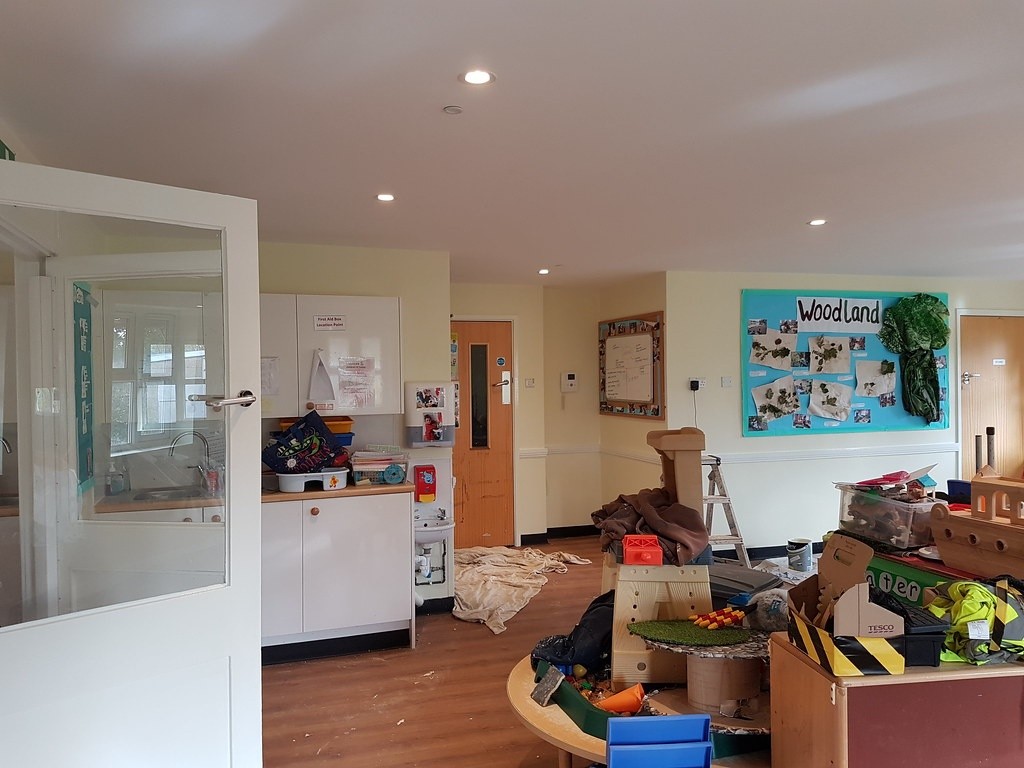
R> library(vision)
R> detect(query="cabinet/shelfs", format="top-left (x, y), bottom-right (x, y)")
top-left (770, 631), bottom-right (1024, 768)
top-left (91, 290), bottom-right (405, 423)
top-left (95, 492), bottom-right (416, 651)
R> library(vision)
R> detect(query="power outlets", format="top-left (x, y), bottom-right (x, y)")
top-left (690, 378), bottom-right (706, 388)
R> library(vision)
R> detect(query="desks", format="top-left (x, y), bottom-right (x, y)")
top-left (507, 653), bottom-right (771, 768)
top-left (823, 531), bottom-right (985, 607)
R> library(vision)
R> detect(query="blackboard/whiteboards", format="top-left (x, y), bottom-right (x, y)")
top-left (605, 333), bottom-right (653, 404)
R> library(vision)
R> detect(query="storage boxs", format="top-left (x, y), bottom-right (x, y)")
top-left (279, 416), bottom-right (355, 433)
top-left (838, 485), bottom-right (949, 549)
top-left (276, 467), bottom-right (350, 493)
top-left (869, 587), bottom-right (951, 667)
top-left (348, 459), bottom-right (409, 487)
top-left (709, 564), bottom-right (783, 612)
top-left (270, 430), bottom-right (355, 446)
top-left (787, 534), bottom-right (905, 677)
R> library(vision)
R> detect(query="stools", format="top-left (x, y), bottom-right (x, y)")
top-left (601, 529), bottom-right (714, 692)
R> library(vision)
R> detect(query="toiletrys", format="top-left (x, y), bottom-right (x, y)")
top-left (103, 459), bottom-right (130, 496)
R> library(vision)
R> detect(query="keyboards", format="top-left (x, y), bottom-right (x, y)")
top-left (903, 601), bottom-right (950, 632)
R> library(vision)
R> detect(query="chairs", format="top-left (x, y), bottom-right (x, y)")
top-left (606, 713), bottom-right (713, 768)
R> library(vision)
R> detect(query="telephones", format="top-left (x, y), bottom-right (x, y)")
top-left (560, 372), bottom-right (578, 393)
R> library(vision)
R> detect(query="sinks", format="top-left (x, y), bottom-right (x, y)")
top-left (132, 483), bottom-right (202, 504)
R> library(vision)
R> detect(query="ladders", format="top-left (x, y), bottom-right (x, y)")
top-left (701, 451), bottom-right (753, 572)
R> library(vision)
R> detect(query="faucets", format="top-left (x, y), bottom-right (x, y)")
top-left (169, 431), bottom-right (209, 469)
